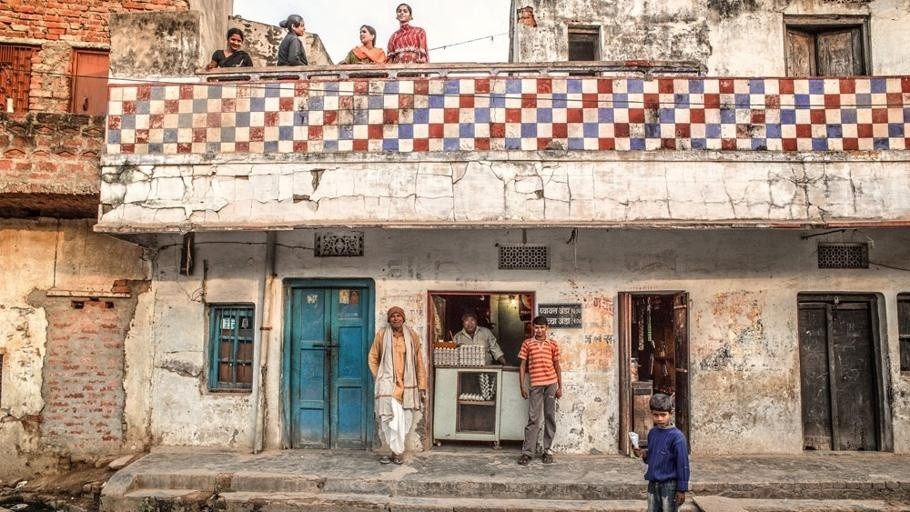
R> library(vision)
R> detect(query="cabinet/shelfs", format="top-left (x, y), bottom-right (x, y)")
top-left (432, 364), bottom-right (530, 450)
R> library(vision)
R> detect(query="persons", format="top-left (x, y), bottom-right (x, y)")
top-left (518, 316), bottom-right (563, 465)
top-left (453, 307), bottom-right (506, 365)
top-left (386, 3), bottom-right (429, 64)
top-left (633, 393), bottom-right (690, 512)
top-left (334, 25), bottom-right (387, 64)
top-left (205, 28), bottom-right (253, 70)
top-left (276, 14), bottom-right (309, 66)
top-left (368, 305), bottom-right (426, 466)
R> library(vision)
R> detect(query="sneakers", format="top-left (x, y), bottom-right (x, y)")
top-left (543, 453), bottom-right (553, 463)
top-left (380, 455), bottom-right (390, 463)
top-left (393, 454), bottom-right (402, 464)
top-left (518, 455), bottom-right (528, 464)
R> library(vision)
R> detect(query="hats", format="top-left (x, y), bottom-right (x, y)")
top-left (461, 308), bottom-right (478, 321)
top-left (387, 307), bottom-right (406, 323)
